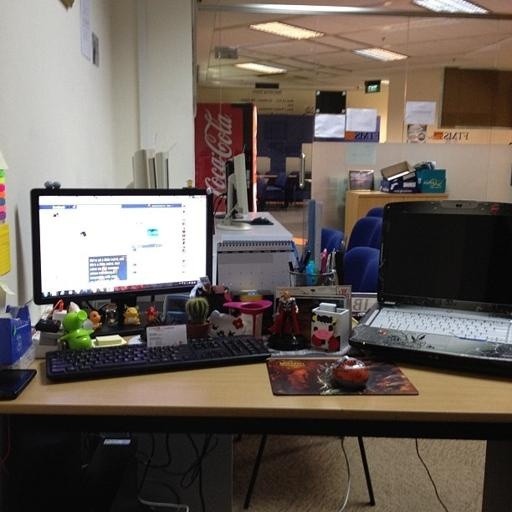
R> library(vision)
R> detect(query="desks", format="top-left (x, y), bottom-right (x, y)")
top-left (258, 174), bottom-right (296, 178)
top-left (1, 314), bottom-right (512, 512)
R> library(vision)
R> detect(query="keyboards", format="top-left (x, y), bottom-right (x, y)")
top-left (45, 334), bottom-right (270, 382)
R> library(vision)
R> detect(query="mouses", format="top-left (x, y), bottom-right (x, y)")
top-left (254, 217), bottom-right (270, 225)
top-left (334, 356), bottom-right (368, 388)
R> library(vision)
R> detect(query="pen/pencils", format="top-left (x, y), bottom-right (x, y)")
top-left (289, 240), bottom-right (336, 273)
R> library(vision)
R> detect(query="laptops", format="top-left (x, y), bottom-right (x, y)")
top-left (349, 200), bottom-right (512, 364)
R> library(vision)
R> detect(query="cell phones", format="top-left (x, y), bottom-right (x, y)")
top-left (1, 367), bottom-right (36, 400)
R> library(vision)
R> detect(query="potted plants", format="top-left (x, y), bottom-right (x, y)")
top-left (187, 298), bottom-right (210, 338)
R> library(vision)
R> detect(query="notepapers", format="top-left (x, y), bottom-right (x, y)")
top-left (96, 335), bottom-right (121, 345)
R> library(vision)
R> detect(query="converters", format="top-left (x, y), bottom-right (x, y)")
top-left (51, 309), bottom-right (67, 330)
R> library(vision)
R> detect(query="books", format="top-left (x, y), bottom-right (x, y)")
top-left (132, 149), bottom-right (169, 190)
top-left (380, 161), bottom-right (410, 181)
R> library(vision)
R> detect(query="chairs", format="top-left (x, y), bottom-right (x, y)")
top-left (263, 173), bottom-right (288, 208)
top-left (321, 208), bottom-right (385, 291)
top-left (243, 247), bottom-right (380, 506)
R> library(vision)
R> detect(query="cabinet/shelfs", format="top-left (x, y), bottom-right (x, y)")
top-left (211, 211), bottom-right (295, 296)
top-left (345, 190), bottom-right (448, 249)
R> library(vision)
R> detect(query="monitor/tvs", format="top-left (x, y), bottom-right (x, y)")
top-left (215, 158), bottom-right (243, 219)
top-left (300, 199), bottom-right (323, 273)
top-left (30, 188), bottom-right (214, 335)
top-left (215, 153), bottom-right (251, 231)
